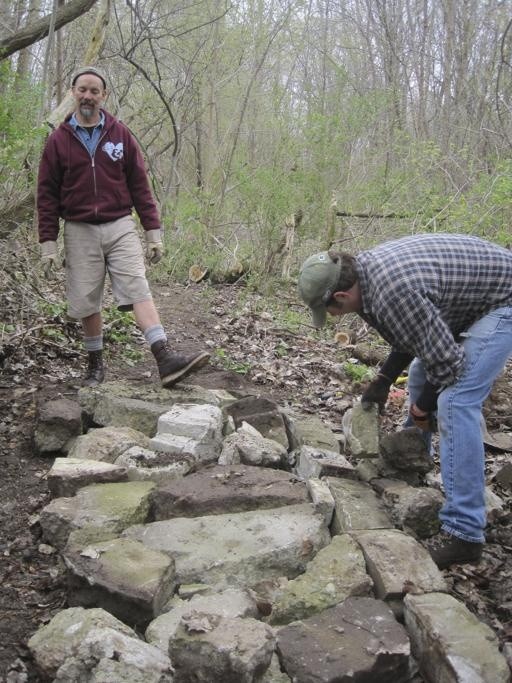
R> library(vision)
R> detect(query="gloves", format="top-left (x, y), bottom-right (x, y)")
top-left (361, 375), bottom-right (392, 416)
top-left (408, 404), bottom-right (438, 433)
top-left (38, 241), bottom-right (61, 281)
top-left (144, 229), bottom-right (164, 265)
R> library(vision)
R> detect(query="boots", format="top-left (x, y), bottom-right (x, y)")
top-left (151, 339), bottom-right (212, 388)
top-left (81, 349), bottom-right (104, 387)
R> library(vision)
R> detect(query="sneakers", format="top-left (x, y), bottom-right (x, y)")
top-left (417, 528), bottom-right (483, 571)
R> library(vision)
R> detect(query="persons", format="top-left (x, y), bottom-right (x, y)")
top-left (292, 233), bottom-right (512, 570)
top-left (35, 65), bottom-right (212, 391)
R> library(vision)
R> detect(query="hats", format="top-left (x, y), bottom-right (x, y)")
top-left (298, 249), bottom-right (342, 330)
top-left (69, 66), bottom-right (107, 90)
